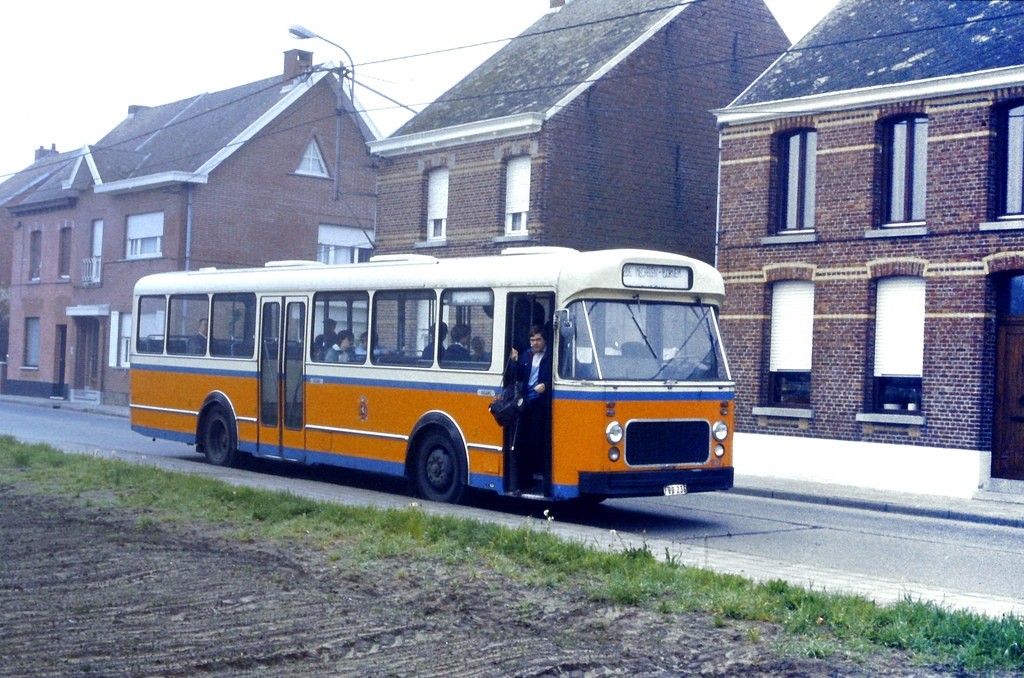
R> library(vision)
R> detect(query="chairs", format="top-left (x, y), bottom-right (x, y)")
top-left (230, 336), bottom-right (249, 356)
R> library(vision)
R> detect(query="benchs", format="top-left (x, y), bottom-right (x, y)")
top-left (167, 335), bottom-right (202, 354)
top-left (146, 335), bottom-right (164, 353)
top-left (377, 349), bottom-right (423, 365)
top-left (186, 338), bottom-right (229, 356)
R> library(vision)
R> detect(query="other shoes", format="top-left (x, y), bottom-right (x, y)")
top-left (512, 488), bottom-right (526, 496)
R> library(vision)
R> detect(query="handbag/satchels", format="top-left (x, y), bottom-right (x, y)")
top-left (488, 351), bottom-right (526, 426)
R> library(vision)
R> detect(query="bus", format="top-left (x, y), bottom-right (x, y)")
top-left (130, 246), bottom-right (736, 509)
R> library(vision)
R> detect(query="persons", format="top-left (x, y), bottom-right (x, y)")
top-left (188, 318), bottom-right (216, 354)
top-left (511, 324), bottom-right (552, 497)
top-left (355, 331), bottom-right (392, 355)
top-left (471, 336), bottom-right (491, 362)
top-left (324, 331), bottom-right (354, 363)
top-left (445, 324), bottom-right (471, 361)
top-left (514, 299), bottom-right (545, 348)
top-left (422, 322), bottom-right (448, 360)
top-left (315, 319), bottom-right (337, 346)
